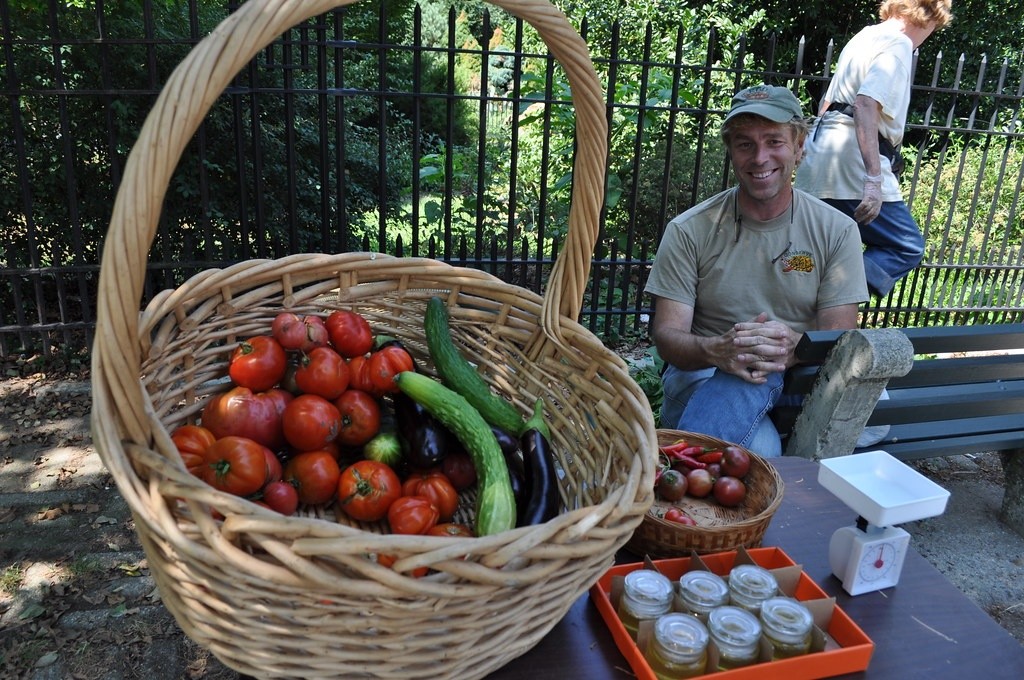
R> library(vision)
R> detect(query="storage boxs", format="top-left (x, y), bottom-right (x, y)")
top-left (587, 546), bottom-right (875, 680)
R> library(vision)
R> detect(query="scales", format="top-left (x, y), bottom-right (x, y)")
top-left (816, 448), bottom-right (954, 599)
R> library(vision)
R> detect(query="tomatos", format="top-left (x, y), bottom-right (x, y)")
top-left (168, 307), bottom-right (475, 579)
top-left (657, 446), bottom-right (750, 526)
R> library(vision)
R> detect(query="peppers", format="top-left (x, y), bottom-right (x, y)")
top-left (654, 439), bottom-right (723, 484)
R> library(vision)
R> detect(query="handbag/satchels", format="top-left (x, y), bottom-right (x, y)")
top-left (813, 101), bottom-right (910, 180)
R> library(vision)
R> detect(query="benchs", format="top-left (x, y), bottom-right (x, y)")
top-left (768, 323), bottom-right (1024, 531)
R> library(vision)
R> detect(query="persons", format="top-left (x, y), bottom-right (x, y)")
top-left (644, 85), bottom-right (870, 459)
top-left (793, 0), bottom-right (953, 297)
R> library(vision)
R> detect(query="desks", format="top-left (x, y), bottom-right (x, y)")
top-left (487, 454), bottom-right (1024, 680)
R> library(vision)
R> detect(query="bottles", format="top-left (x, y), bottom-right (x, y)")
top-left (759, 596), bottom-right (814, 662)
top-left (729, 565), bottom-right (778, 618)
top-left (678, 570), bottom-right (729, 626)
top-left (645, 612), bottom-right (709, 680)
top-left (617, 569), bottom-right (675, 643)
top-left (707, 606), bottom-right (763, 672)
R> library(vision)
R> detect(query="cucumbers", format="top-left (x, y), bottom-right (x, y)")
top-left (422, 298), bottom-right (525, 434)
top-left (393, 372), bottom-right (517, 536)
top-left (364, 424), bottom-right (402, 465)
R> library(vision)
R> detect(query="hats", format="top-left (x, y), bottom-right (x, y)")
top-left (721, 85), bottom-right (802, 128)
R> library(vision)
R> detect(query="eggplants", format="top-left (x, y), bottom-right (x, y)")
top-left (371, 336), bottom-right (558, 530)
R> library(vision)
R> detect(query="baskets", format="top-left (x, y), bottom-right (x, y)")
top-left (636, 428), bottom-right (784, 551)
top-left (91, 0), bottom-right (654, 680)
